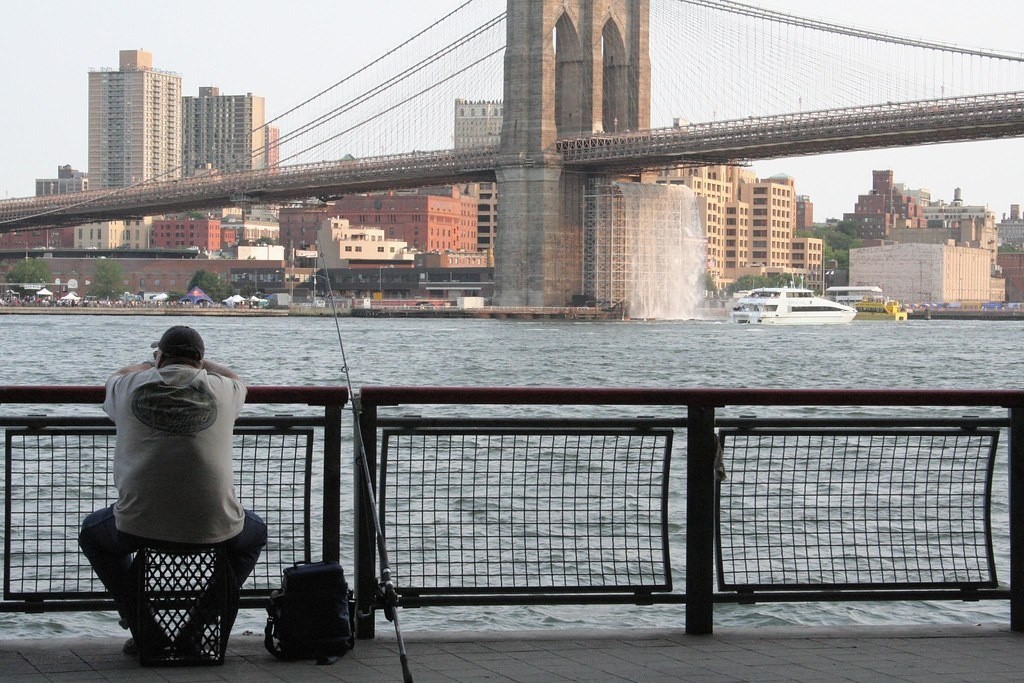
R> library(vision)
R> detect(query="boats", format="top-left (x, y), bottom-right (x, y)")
top-left (818, 282), bottom-right (888, 314)
top-left (728, 266), bottom-right (858, 326)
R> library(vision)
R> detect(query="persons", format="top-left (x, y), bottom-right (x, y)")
top-left (79, 326), bottom-right (268, 662)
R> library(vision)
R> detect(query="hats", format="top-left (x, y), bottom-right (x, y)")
top-left (150, 325), bottom-right (206, 360)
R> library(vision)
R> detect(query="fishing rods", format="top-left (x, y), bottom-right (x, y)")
top-left (316, 228), bottom-right (415, 683)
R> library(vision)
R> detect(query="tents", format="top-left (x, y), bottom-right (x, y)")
top-left (178, 286), bottom-right (212, 304)
top-left (223, 295), bottom-right (267, 306)
top-left (153, 293), bottom-right (170, 300)
top-left (36, 288), bottom-right (54, 307)
top-left (61, 293), bottom-right (80, 300)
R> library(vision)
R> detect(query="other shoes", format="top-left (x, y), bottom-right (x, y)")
top-left (122, 631), bottom-right (172, 656)
top-left (173, 621), bottom-right (204, 653)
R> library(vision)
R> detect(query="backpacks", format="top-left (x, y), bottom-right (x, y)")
top-left (263, 560), bottom-right (357, 665)
